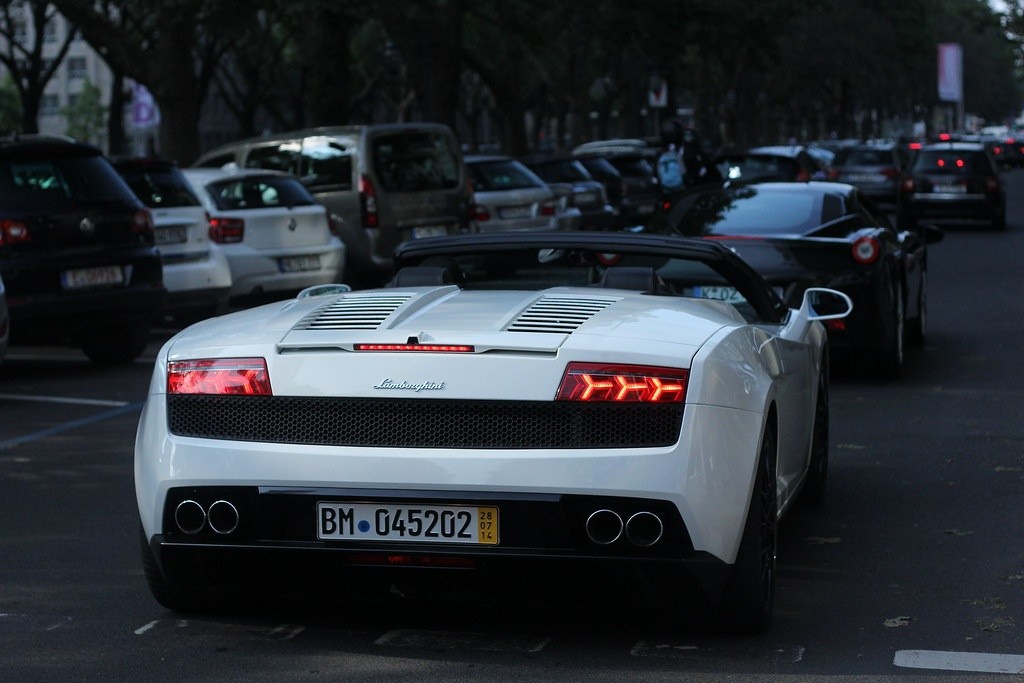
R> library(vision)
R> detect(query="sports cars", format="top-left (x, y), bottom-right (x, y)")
top-left (134, 231), bottom-right (855, 633)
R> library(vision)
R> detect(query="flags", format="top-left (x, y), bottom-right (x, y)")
top-left (130, 83), bottom-right (162, 129)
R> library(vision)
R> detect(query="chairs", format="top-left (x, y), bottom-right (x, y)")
top-left (600, 267), bottom-right (658, 295)
top-left (393, 267), bottom-right (453, 288)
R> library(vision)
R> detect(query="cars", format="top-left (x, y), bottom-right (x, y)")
top-left (494, 137), bottom-right (819, 232)
top-left (604, 179), bottom-right (944, 371)
top-left (176, 168), bottom-right (345, 297)
top-left (936, 126), bottom-right (1024, 165)
top-left (896, 144), bottom-right (1006, 230)
top-left (831, 145), bottom-right (912, 211)
top-left (461, 155), bottom-right (558, 269)
top-left (104, 156), bottom-right (232, 311)
top-left (0, 133), bottom-right (169, 365)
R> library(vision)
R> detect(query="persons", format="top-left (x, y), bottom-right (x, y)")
top-left (657, 126), bottom-right (713, 192)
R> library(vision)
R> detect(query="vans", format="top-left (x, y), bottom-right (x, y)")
top-left (189, 123), bottom-right (477, 280)
